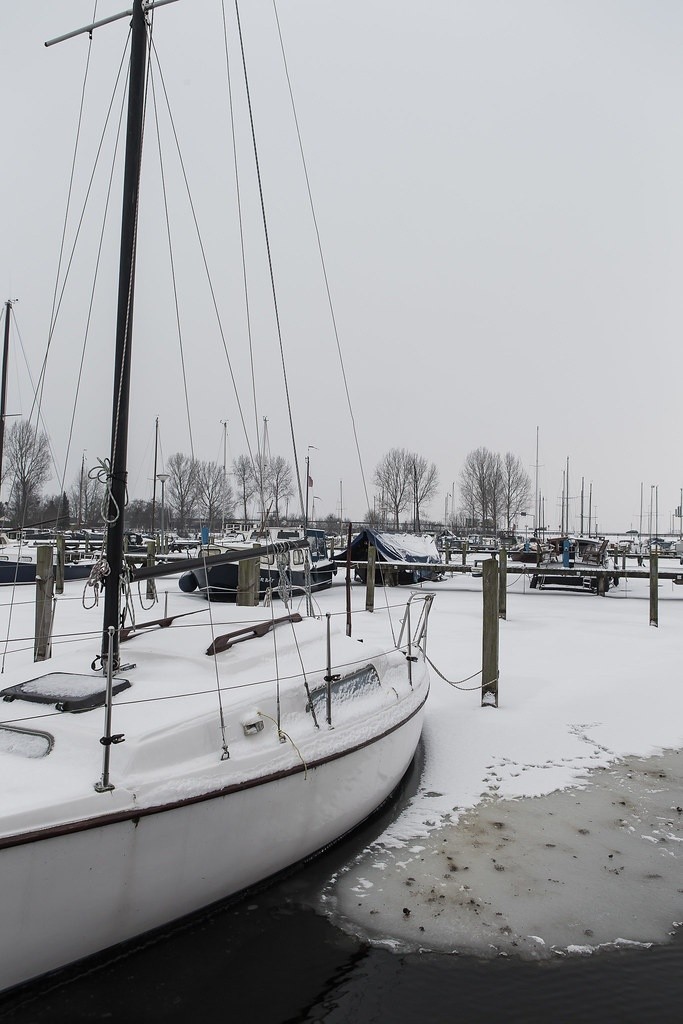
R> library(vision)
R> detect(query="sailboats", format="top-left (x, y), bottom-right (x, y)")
top-left (0, 299), bottom-right (683, 603)
top-left (1, 0), bottom-right (435, 995)
